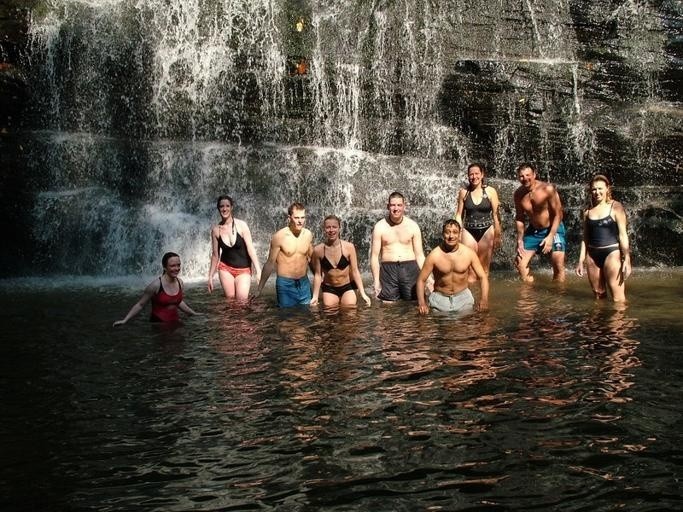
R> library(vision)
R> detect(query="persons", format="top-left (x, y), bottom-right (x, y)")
top-left (304, 213), bottom-right (370, 307)
top-left (367, 190), bottom-right (434, 305)
top-left (575, 174), bottom-right (631, 303)
top-left (204, 193), bottom-right (261, 300)
top-left (246, 202), bottom-right (317, 309)
top-left (109, 252), bottom-right (206, 328)
top-left (455, 163), bottom-right (501, 282)
top-left (512, 163), bottom-right (566, 281)
top-left (414, 216), bottom-right (489, 315)
